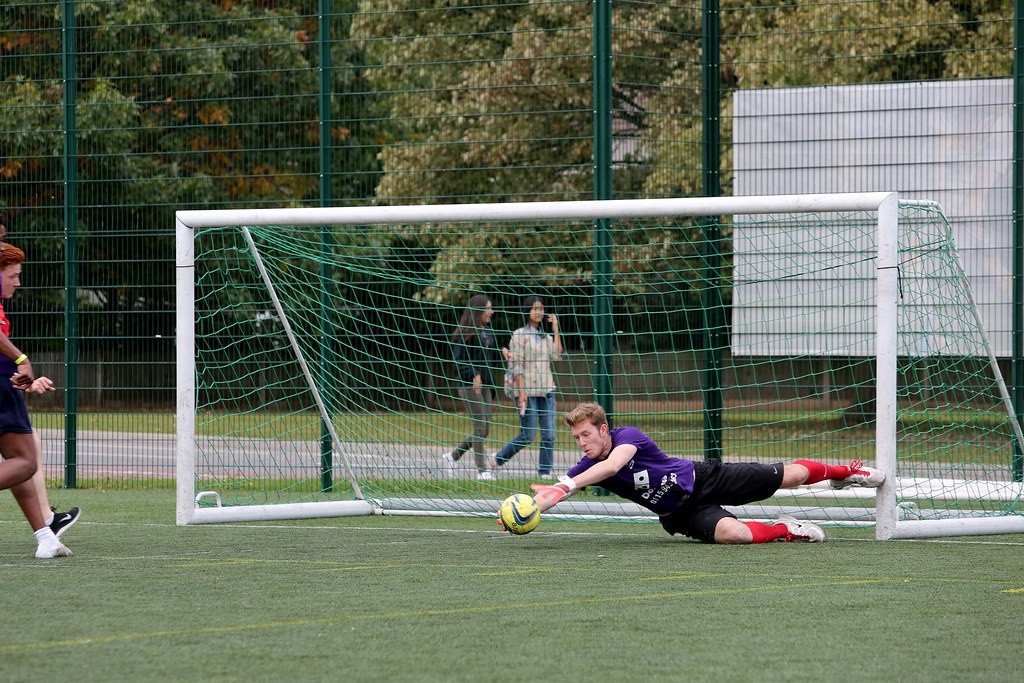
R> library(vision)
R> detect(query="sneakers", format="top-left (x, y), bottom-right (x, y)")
top-left (830, 457), bottom-right (886, 490)
top-left (47, 506), bottom-right (81, 537)
top-left (35, 536), bottom-right (73, 558)
top-left (774, 513), bottom-right (826, 542)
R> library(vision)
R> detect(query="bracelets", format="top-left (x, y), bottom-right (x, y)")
top-left (15, 354), bottom-right (27, 364)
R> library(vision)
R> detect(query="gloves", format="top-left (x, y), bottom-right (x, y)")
top-left (497, 510), bottom-right (513, 536)
top-left (530, 475), bottom-right (577, 513)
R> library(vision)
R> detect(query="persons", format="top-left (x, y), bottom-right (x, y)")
top-left (486, 295), bottom-right (562, 480)
top-left (441, 295), bottom-right (512, 481)
top-left (0, 225), bottom-right (82, 559)
top-left (496, 402), bottom-right (886, 545)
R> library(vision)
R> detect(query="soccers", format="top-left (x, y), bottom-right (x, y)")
top-left (500, 493), bottom-right (541, 535)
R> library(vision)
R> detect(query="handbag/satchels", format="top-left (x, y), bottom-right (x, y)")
top-left (504, 328), bottom-right (525, 398)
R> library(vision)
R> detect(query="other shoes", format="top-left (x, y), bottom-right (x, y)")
top-left (442, 453), bottom-right (459, 479)
top-left (540, 474), bottom-right (553, 480)
top-left (477, 471), bottom-right (496, 481)
top-left (488, 453), bottom-right (497, 475)
